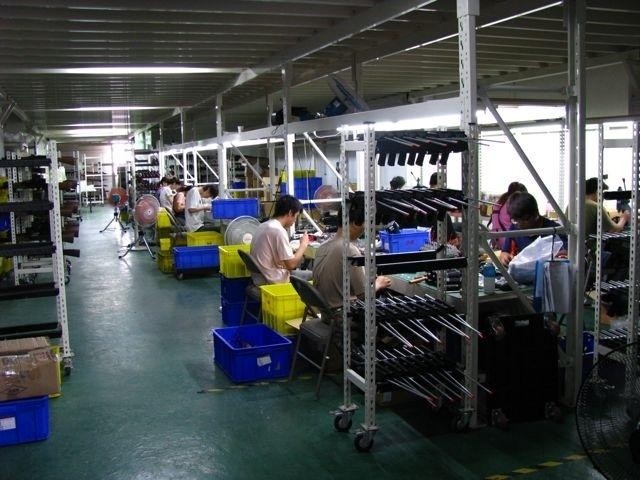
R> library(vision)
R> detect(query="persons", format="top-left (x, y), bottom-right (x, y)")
top-left (312, 208), bottom-right (392, 345)
top-left (429, 172), bottom-right (630, 406)
top-left (153, 171), bottom-right (221, 232)
top-left (251, 195), bottom-right (312, 298)
top-left (390, 177), bottom-right (405, 190)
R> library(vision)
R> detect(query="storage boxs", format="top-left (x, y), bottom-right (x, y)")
top-left (0, 334), bottom-right (62, 402)
top-left (279, 168), bottom-right (323, 210)
top-left (378, 228), bottom-right (432, 255)
top-left (0, 393), bottom-right (52, 448)
top-left (257, 277), bottom-right (316, 340)
top-left (211, 323), bottom-right (293, 384)
top-left (156, 198), bottom-right (261, 325)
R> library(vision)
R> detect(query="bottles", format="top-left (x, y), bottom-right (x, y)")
top-left (482, 258), bottom-right (497, 293)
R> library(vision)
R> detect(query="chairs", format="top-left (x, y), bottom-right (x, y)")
top-left (287, 271), bottom-right (347, 400)
top-left (237, 249), bottom-right (270, 328)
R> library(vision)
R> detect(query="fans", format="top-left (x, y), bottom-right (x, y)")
top-left (312, 183), bottom-right (339, 216)
top-left (573, 339), bottom-right (640, 479)
top-left (119, 193), bottom-right (162, 262)
top-left (324, 72), bottom-right (371, 117)
top-left (99, 186), bottom-right (130, 236)
